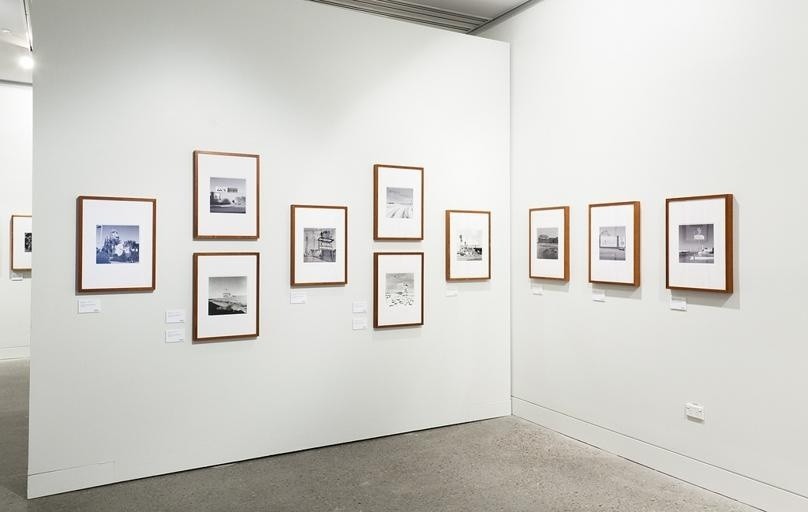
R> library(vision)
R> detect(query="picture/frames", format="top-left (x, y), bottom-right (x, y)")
top-left (372, 164), bottom-right (425, 241)
top-left (372, 252), bottom-right (425, 329)
top-left (445, 209), bottom-right (492, 281)
top-left (192, 251), bottom-right (260, 340)
top-left (11, 215), bottom-right (32, 272)
top-left (588, 201), bottom-right (641, 287)
top-left (191, 150), bottom-right (261, 241)
top-left (290, 204), bottom-right (348, 288)
top-left (665, 193), bottom-right (734, 294)
top-left (75, 194), bottom-right (157, 293)
top-left (528, 206), bottom-right (572, 281)
